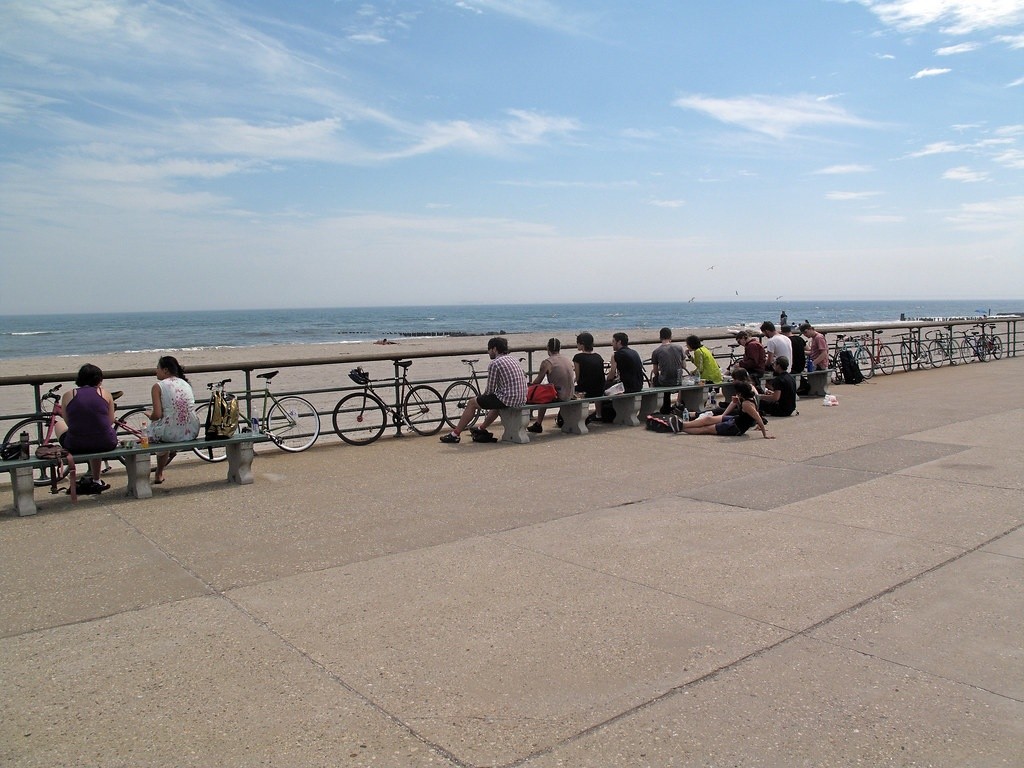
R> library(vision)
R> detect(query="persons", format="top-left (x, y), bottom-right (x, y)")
top-left (669, 321), bottom-right (828, 440)
top-left (652, 328), bottom-right (686, 415)
top-left (56, 364), bottom-right (118, 486)
top-left (527, 338), bottom-right (574, 433)
top-left (590, 333), bottom-right (643, 421)
top-left (144, 356), bottom-right (200, 484)
top-left (554, 333), bottom-right (605, 427)
top-left (440, 337), bottom-right (528, 443)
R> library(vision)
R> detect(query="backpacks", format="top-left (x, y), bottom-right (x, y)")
top-left (204, 389), bottom-right (240, 459)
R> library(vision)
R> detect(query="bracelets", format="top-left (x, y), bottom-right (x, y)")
top-left (689, 355), bottom-right (691, 358)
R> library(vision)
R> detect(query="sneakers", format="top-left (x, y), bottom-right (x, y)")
top-left (468, 427), bottom-right (479, 435)
top-left (669, 415), bottom-right (683, 434)
top-left (440, 434), bottom-right (460, 443)
top-left (527, 422), bottom-right (542, 433)
top-left (589, 415), bottom-right (603, 424)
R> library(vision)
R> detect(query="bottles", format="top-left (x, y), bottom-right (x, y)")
top-left (252, 417), bottom-right (259, 436)
top-left (20, 431), bottom-right (30, 460)
top-left (710, 389), bottom-right (718, 407)
top-left (694, 370), bottom-right (701, 385)
top-left (682, 408), bottom-right (689, 422)
top-left (807, 359), bottom-right (813, 372)
top-left (140, 422), bottom-right (149, 448)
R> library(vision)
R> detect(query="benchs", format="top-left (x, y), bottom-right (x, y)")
top-left (0, 432), bottom-right (268, 517)
top-left (500, 369), bottom-right (837, 444)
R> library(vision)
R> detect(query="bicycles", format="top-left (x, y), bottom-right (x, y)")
top-left (1, 384), bottom-right (177, 487)
top-left (603, 323), bottom-right (1003, 387)
top-left (332, 360), bottom-right (446, 445)
top-left (442, 357), bottom-right (526, 430)
top-left (193, 370), bottom-right (320, 463)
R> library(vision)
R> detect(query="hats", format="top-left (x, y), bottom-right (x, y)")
top-left (799, 323), bottom-right (811, 337)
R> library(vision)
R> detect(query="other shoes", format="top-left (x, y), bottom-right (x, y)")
top-left (154, 478), bottom-right (165, 484)
top-left (672, 401), bottom-right (685, 419)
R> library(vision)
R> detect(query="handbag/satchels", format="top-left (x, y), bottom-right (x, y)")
top-left (66, 476), bottom-right (110, 495)
top-left (36, 445), bottom-right (68, 494)
top-left (646, 413), bottom-right (673, 433)
top-left (526, 383), bottom-right (558, 403)
